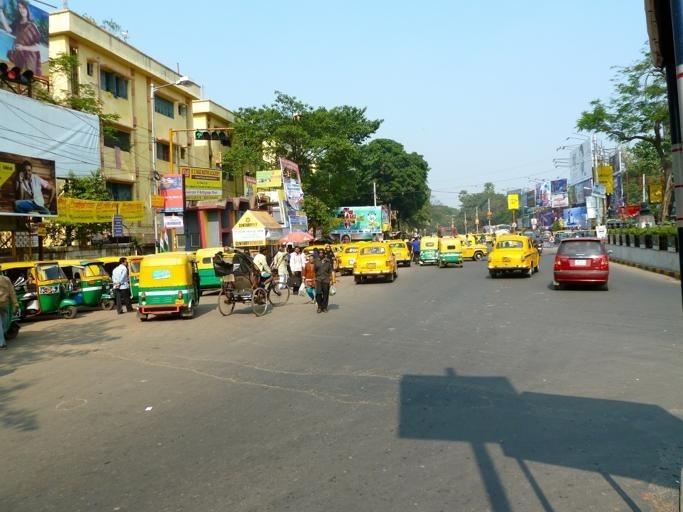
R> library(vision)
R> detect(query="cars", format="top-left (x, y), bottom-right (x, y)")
top-left (516, 230), bottom-right (594, 246)
top-left (485, 235), bottom-right (542, 278)
top-left (637, 212), bottom-right (655, 230)
top-left (305, 232), bottom-right (486, 283)
top-left (551, 237), bottom-right (612, 291)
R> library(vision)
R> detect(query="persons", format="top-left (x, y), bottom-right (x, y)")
top-left (410, 237), bottom-right (420, 261)
top-left (111, 257), bottom-right (138, 314)
top-left (532, 234), bottom-right (543, 248)
top-left (0, 0), bottom-right (42, 77)
top-left (0, 266), bottom-right (19, 350)
top-left (407, 238), bottom-right (411, 252)
top-left (340, 234), bottom-right (350, 244)
top-left (244, 243), bottom-right (338, 314)
top-left (13, 160), bottom-right (56, 213)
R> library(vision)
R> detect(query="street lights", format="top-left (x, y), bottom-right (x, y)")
top-left (583, 186), bottom-right (600, 226)
top-left (524, 204), bottom-right (540, 230)
top-left (463, 221), bottom-right (467, 233)
top-left (474, 217), bottom-right (479, 232)
top-left (449, 223), bottom-right (454, 227)
top-left (486, 211), bottom-right (493, 232)
top-left (149, 74), bottom-right (194, 253)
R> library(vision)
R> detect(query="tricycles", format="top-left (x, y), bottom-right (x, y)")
top-left (212, 249), bottom-right (291, 317)
top-left (0, 246), bottom-right (240, 348)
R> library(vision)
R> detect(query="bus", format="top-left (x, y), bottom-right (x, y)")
top-left (437, 227), bottom-right (457, 238)
top-left (481, 223), bottom-right (510, 232)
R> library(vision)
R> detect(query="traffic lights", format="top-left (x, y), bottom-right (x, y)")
top-left (193, 127), bottom-right (231, 143)
